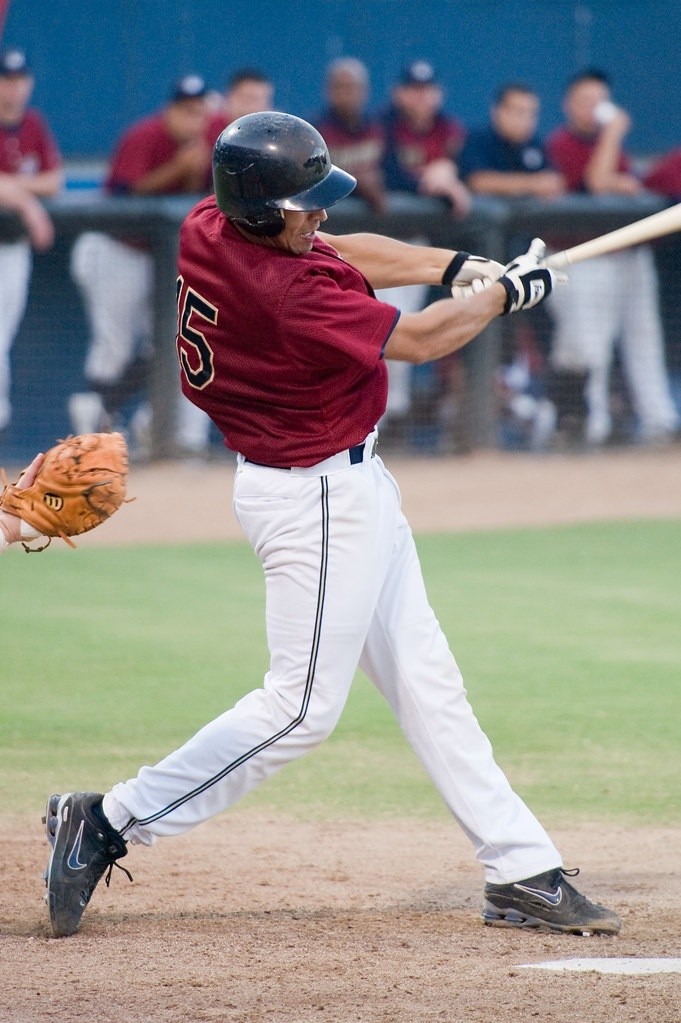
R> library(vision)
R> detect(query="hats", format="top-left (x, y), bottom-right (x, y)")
top-left (400, 61), bottom-right (437, 88)
top-left (173, 75), bottom-right (206, 101)
top-left (2, 51), bottom-right (31, 78)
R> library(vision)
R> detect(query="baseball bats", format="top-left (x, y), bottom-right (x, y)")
top-left (450, 202), bottom-right (681, 299)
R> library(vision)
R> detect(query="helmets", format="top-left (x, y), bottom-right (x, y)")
top-left (213, 111), bottom-right (356, 239)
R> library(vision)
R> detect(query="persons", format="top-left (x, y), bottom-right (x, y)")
top-left (68, 59), bottom-right (681, 452)
top-left (0, 51), bottom-right (65, 430)
top-left (44, 112), bottom-right (621, 937)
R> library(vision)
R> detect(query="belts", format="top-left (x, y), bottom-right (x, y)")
top-left (247, 445), bottom-right (368, 476)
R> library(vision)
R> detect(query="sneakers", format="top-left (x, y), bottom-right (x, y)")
top-left (42, 792), bottom-right (135, 938)
top-left (483, 864), bottom-right (621, 935)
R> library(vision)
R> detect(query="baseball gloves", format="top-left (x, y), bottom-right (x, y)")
top-left (0, 432), bottom-right (130, 538)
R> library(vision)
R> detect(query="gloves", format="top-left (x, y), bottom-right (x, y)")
top-left (497, 237), bottom-right (571, 314)
top-left (440, 250), bottom-right (508, 302)
